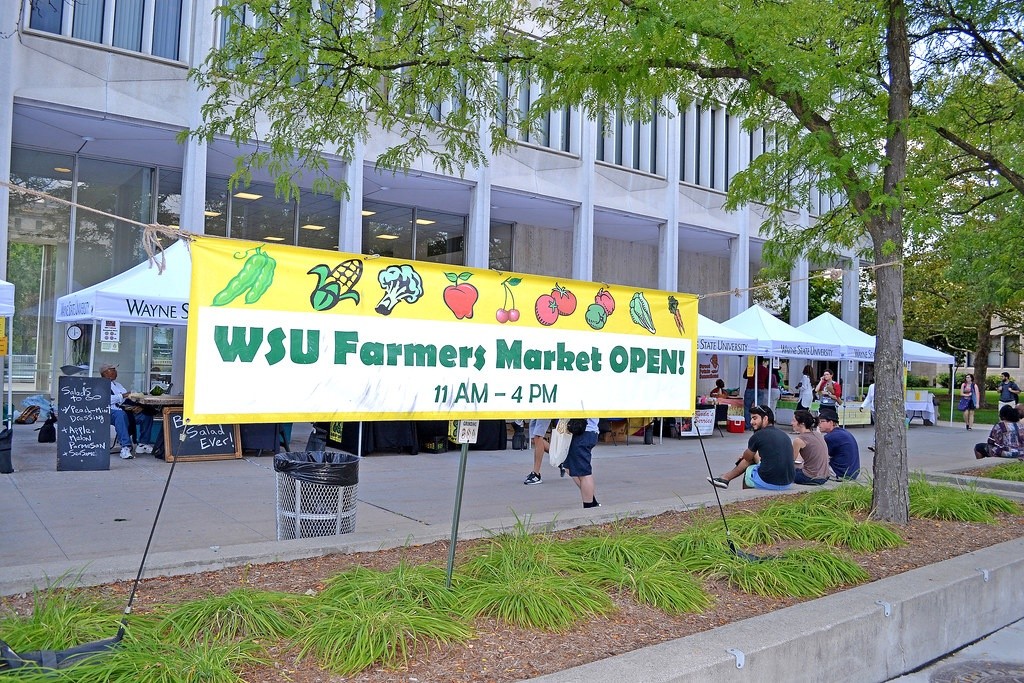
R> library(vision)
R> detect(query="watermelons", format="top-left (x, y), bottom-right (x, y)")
top-left (150, 385), bottom-right (164, 396)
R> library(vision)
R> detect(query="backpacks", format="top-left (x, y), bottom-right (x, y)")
top-left (1000, 382), bottom-right (1018, 405)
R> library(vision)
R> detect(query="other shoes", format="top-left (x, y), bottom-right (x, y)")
top-left (966, 425), bottom-right (971, 431)
top-left (868, 444), bottom-right (875, 451)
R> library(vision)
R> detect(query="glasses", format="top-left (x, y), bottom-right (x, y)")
top-left (756, 405), bottom-right (765, 412)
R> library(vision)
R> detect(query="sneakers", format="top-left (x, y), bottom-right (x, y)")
top-left (707, 476), bottom-right (729, 489)
top-left (135, 443), bottom-right (153, 454)
top-left (120, 447), bottom-right (134, 459)
top-left (524, 471), bottom-right (543, 485)
top-left (558, 463), bottom-right (566, 477)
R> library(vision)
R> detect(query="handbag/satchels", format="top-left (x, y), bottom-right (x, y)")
top-left (567, 418), bottom-right (588, 436)
top-left (957, 397), bottom-right (971, 411)
top-left (971, 383), bottom-right (980, 406)
top-left (549, 420), bottom-right (573, 468)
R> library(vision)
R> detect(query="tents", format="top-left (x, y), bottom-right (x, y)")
top-left (0, 280), bottom-right (15, 430)
top-left (697, 304), bottom-right (956, 423)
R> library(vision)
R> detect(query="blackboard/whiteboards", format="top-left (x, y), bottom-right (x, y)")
top-left (57, 375), bottom-right (111, 471)
top-left (163, 407), bottom-right (243, 462)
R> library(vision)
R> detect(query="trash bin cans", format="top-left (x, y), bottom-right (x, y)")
top-left (275, 451), bottom-right (360, 541)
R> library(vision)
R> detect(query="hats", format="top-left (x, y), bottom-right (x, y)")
top-left (98, 363), bottom-right (119, 374)
top-left (819, 409), bottom-right (839, 421)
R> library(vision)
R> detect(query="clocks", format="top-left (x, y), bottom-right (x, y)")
top-left (67, 325), bottom-right (83, 341)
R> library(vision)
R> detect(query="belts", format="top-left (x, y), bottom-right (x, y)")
top-left (999, 400), bottom-right (1013, 402)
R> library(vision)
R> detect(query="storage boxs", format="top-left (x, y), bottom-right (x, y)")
top-left (602, 422), bottom-right (627, 442)
top-left (727, 416), bottom-right (745, 433)
top-left (419, 436), bottom-right (450, 455)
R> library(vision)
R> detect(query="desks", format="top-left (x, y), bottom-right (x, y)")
top-left (696, 404), bottom-right (728, 437)
top-left (837, 401), bottom-right (871, 427)
top-left (905, 403), bottom-right (934, 425)
top-left (128, 394), bottom-right (183, 406)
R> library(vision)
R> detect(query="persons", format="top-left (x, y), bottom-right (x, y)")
top-left (960, 374), bottom-right (979, 431)
top-left (524, 418), bottom-right (602, 508)
top-left (997, 372), bottom-right (1022, 421)
top-left (815, 369), bottom-right (843, 412)
top-left (710, 379), bottom-right (727, 397)
top-left (818, 409), bottom-right (861, 482)
top-left (974, 403), bottom-right (1024, 459)
top-left (99, 363), bottom-right (153, 459)
top-left (743, 356), bottom-right (781, 429)
top-left (859, 383), bottom-right (875, 451)
top-left (789, 365), bottom-right (814, 434)
top-left (791, 410), bottom-right (830, 486)
top-left (707, 405), bottom-right (794, 490)
